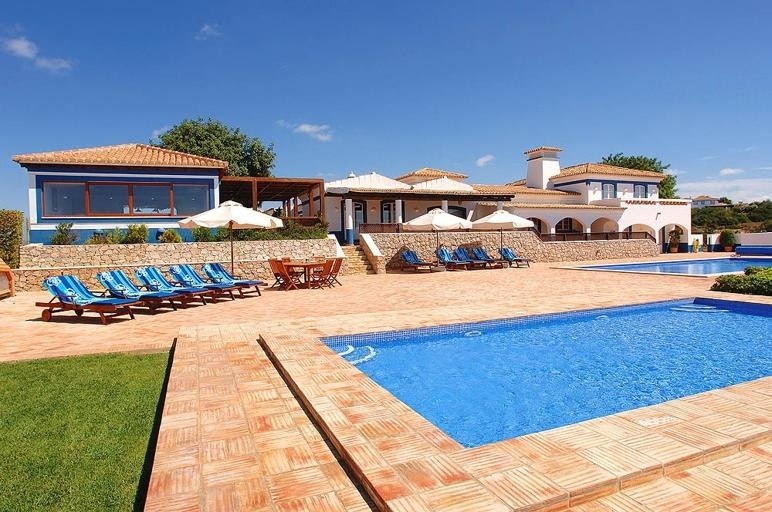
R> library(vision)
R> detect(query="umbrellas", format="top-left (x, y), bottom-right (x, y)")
top-left (177, 200), bottom-right (285, 279)
top-left (402, 206), bottom-right (474, 266)
top-left (470, 208), bottom-right (534, 260)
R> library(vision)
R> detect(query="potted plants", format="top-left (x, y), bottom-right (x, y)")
top-left (668, 229), bottom-right (681, 253)
top-left (719, 230), bottom-right (736, 252)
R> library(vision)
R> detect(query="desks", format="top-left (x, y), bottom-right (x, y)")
top-left (282, 260), bottom-right (326, 289)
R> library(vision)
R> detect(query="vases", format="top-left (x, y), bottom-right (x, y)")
top-left (702, 246), bottom-right (708, 252)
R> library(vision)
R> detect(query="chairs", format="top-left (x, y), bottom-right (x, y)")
top-left (320, 258), bottom-right (342, 287)
top-left (268, 259), bottom-right (292, 288)
top-left (136, 268), bottom-right (212, 308)
top-left (400, 246), bottom-right (532, 273)
top-left (37, 276), bottom-right (144, 324)
top-left (282, 257), bottom-right (304, 284)
top-left (168, 264), bottom-right (244, 301)
top-left (96, 269), bottom-right (183, 316)
top-left (309, 260), bottom-right (334, 290)
top-left (276, 261), bottom-right (300, 290)
top-left (201, 262), bottom-right (269, 298)
